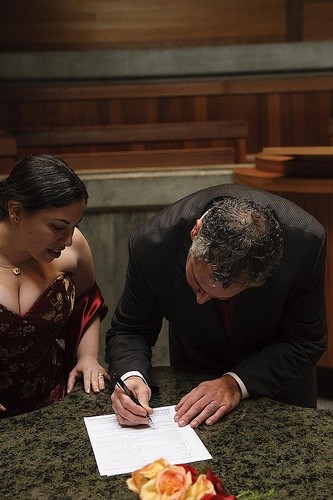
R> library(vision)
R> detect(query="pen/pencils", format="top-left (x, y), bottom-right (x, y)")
top-left (110, 373), bottom-right (153, 424)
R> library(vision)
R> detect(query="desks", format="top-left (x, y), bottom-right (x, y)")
top-left (0, 366), bottom-right (333, 500)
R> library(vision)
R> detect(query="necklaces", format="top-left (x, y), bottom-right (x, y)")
top-left (0, 260), bottom-right (29, 275)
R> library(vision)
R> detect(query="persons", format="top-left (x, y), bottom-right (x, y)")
top-left (105, 183), bottom-right (329, 428)
top-left (0, 154), bottom-right (112, 419)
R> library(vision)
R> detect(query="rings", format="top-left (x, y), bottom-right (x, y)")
top-left (211, 402), bottom-right (216, 412)
top-left (98, 372), bottom-right (104, 378)
